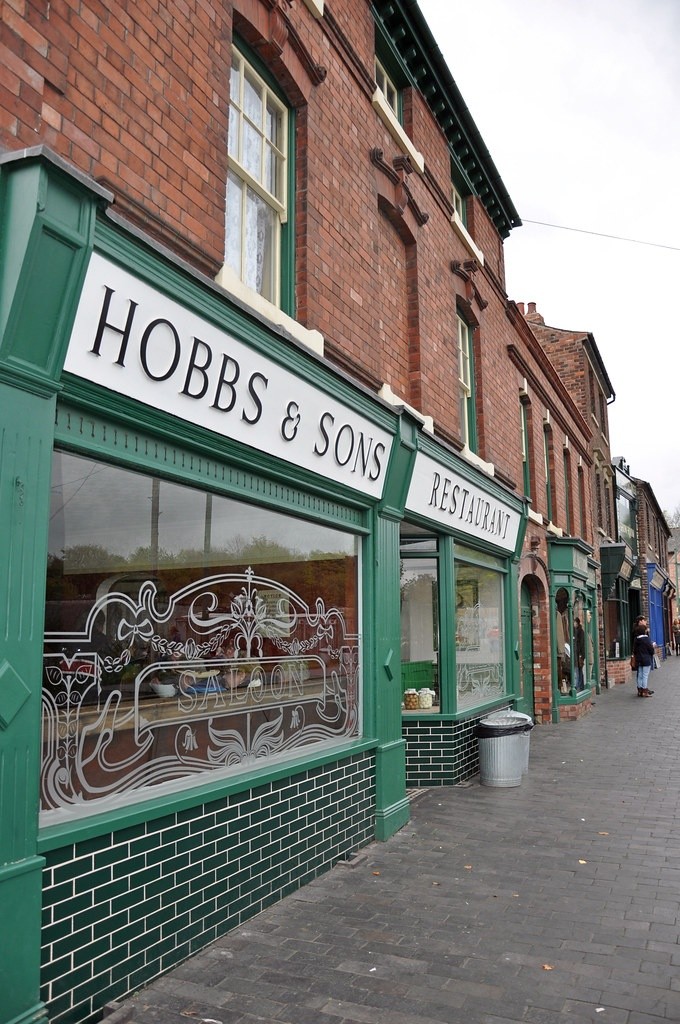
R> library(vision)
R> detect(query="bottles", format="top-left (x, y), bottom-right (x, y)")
top-left (418, 687), bottom-right (436, 709)
top-left (404, 689), bottom-right (418, 711)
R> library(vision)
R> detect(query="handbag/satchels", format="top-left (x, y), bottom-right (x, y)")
top-left (579, 655), bottom-right (584, 667)
top-left (630, 656), bottom-right (638, 668)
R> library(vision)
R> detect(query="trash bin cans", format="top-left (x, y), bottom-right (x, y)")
top-left (477, 715), bottom-right (529, 786)
top-left (487, 710), bottom-right (534, 776)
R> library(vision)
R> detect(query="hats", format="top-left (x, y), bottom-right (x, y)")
top-left (574, 617), bottom-right (580, 624)
top-left (635, 615), bottom-right (646, 620)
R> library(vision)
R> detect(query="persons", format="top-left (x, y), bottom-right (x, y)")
top-left (150, 642), bottom-right (207, 686)
top-left (587, 610), bottom-right (595, 705)
top-left (222, 651), bottom-right (261, 690)
top-left (630, 616), bottom-right (659, 698)
top-left (53, 607), bottom-right (135, 704)
top-left (672, 618), bottom-right (680, 656)
top-left (274, 654), bottom-right (310, 682)
top-left (573, 618), bottom-right (586, 691)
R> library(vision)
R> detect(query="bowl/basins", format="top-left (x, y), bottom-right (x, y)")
top-left (150, 683), bottom-right (179, 698)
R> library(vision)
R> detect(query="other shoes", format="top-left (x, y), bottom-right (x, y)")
top-left (649, 691), bottom-right (654, 694)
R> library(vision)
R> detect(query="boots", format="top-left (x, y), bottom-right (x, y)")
top-left (638, 688), bottom-right (642, 696)
top-left (642, 688), bottom-right (652, 697)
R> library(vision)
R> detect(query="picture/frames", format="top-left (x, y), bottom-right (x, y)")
top-left (432, 579), bottom-right (481, 652)
